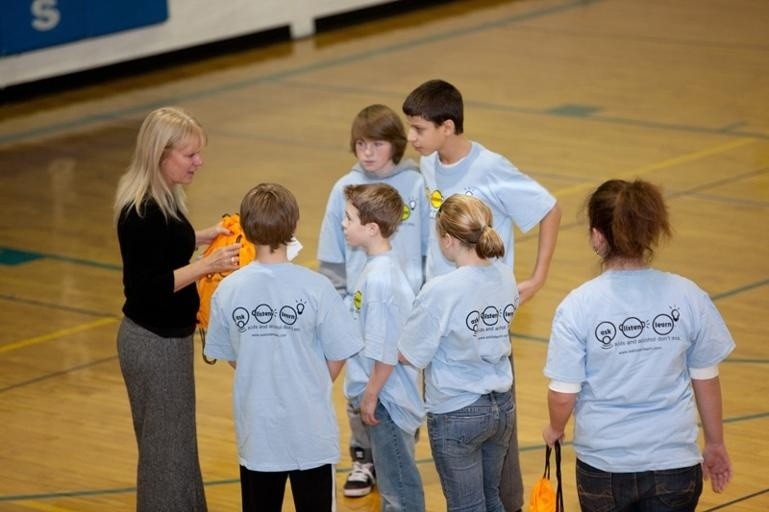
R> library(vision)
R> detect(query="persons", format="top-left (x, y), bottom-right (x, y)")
top-left (204, 182), bottom-right (365, 512)
top-left (403, 80), bottom-right (561, 512)
top-left (116, 107), bottom-right (241, 512)
top-left (397, 196), bottom-right (519, 512)
top-left (317, 104), bottom-right (425, 498)
top-left (542, 179), bottom-right (736, 512)
top-left (343, 182), bottom-right (427, 512)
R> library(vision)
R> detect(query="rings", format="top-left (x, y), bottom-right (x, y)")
top-left (231, 256), bottom-right (235, 263)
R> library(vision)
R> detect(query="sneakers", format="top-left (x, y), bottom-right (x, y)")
top-left (344, 446), bottom-right (375, 497)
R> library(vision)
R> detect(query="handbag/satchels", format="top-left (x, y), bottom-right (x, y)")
top-left (191, 212), bottom-right (257, 365)
top-left (529, 438), bottom-right (564, 512)
